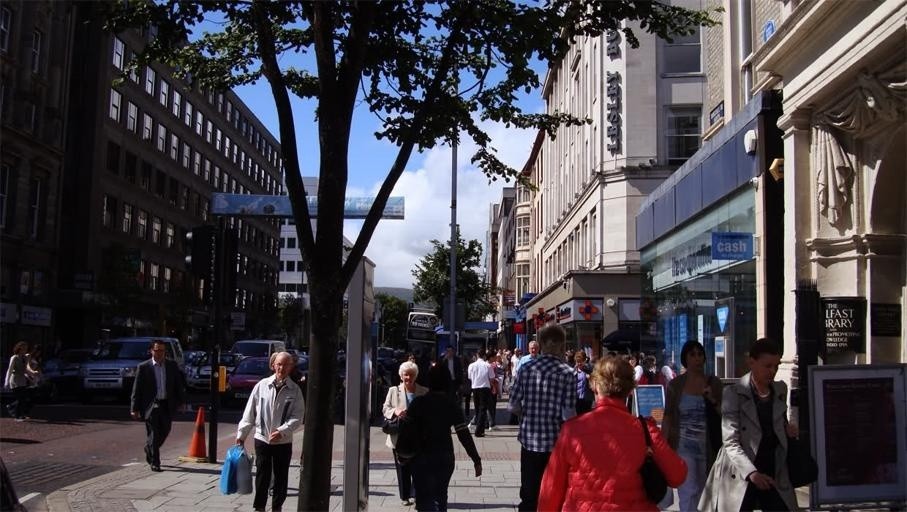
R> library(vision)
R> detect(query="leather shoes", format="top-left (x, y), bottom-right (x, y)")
top-left (144, 446), bottom-right (162, 472)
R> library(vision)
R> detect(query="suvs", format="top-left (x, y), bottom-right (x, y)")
top-left (84, 336), bottom-right (188, 406)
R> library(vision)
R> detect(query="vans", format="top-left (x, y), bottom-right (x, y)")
top-left (229, 340), bottom-right (284, 359)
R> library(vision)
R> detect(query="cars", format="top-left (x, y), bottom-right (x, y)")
top-left (183, 344), bottom-right (345, 407)
top-left (374, 346), bottom-right (405, 420)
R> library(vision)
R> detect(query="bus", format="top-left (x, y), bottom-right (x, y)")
top-left (406, 305), bottom-right (439, 374)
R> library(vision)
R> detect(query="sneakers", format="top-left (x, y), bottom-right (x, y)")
top-left (402, 498), bottom-right (415, 506)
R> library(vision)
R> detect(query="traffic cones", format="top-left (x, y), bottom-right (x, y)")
top-left (187, 406), bottom-right (207, 458)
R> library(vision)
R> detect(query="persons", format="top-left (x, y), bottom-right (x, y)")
top-left (717, 337), bottom-right (801, 510)
top-left (1, 338), bottom-right (47, 421)
top-left (390, 345), bottom-right (523, 437)
top-left (659, 341), bottom-right (724, 512)
top-left (516, 340), bottom-right (540, 372)
top-left (660, 356), bottom-right (677, 384)
top-left (130, 341), bottom-right (186, 474)
top-left (533, 355), bottom-right (688, 512)
top-left (236, 350), bottom-right (306, 511)
top-left (507, 325), bottom-right (578, 511)
top-left (382, 361), bottom-right (482, 512)
top-left (565, 347), bottom-right (656, 416)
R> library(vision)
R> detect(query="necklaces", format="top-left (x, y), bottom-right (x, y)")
top-left (753, 382), bottom-right (769, 396)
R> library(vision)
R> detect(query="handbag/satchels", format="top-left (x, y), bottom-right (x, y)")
top-left (640, 454), bottom-right (681, 511)
top-left (786, 435), bottom-right (819, 488)
top-left (236, 449), bottom-right (254, 494)
top-left (496, 367), bottom-right (504, 375)
top-left (383, 415), bottom-right (401, 435)
